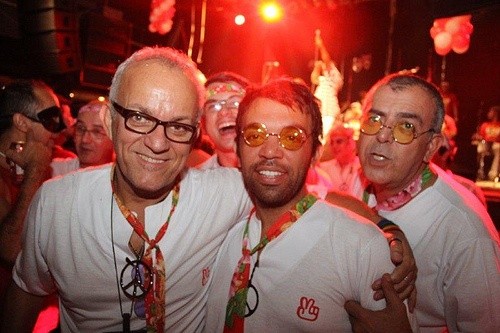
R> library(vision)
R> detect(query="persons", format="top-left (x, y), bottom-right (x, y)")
top-left (0, 33), bottom-right (500, 333)
top-left (204, 78), bottom-right (419, 333)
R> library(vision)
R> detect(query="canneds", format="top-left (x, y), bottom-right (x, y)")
top-left (8, 141), bottom-right (27, 182)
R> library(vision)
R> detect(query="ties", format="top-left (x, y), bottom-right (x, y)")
top-left (109, 160), bottom-right (180, 333)
top-left (223, 193), bottom-right (318, 333)
top-left (361, 166), bottom-right (439, 214)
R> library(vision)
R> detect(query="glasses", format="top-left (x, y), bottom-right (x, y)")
top-left (205, 98), bottom-right (241, 112)
top-left (359, 114), bottom-right (434, 144)
top-left (74, 124), bottom-right (107, 138)
top-left (242, 123), bottom-right (313, 151)
top-left (112, 101), bottom-right (201, 144)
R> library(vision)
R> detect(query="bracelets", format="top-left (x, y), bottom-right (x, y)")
top-left (375, 218), bottom-right (395, 229)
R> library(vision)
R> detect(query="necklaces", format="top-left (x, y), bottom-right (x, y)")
top-left (120, 240), bottom-right (153, 300)
top-left (244, 251), bottom-right (261, 317)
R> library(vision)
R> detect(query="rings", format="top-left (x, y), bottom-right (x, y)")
top-left (388, 236), bottom-right (403, 246)
top-left (404, 276), bottom-right (411, 284)
